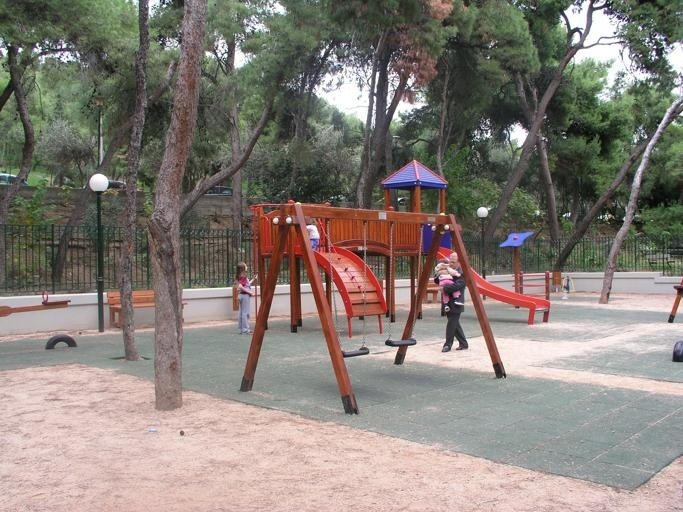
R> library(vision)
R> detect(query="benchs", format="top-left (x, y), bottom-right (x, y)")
top-left (417, 279), bottom-right (443, 303)
top-left (106, 288), bottom-right (186, 330)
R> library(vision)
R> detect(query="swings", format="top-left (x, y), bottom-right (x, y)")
top-left (386, 221), bottom-right (424, 347)
top-left (325, 216), bottom-right (370, 358)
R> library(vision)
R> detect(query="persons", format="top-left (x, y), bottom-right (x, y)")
top-left (306, 219), bottom-right (320, 250)
top-left (235, 261), bottom-right (257, 334)
top-left (434, 252), bottom-right (468, 352)
top-left (434, 259), bottom-right (464, 312)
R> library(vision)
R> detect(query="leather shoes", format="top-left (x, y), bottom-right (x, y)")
top-left (442, 345), bottom-right (468, 352)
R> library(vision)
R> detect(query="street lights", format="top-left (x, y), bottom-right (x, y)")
top-left (88, 174), bottom-right (109, 333)
top-left (476, 206), bottom-right (488, 301)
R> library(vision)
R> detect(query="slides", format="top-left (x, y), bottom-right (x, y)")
top-left (435, 245), bottom-right (551, 312)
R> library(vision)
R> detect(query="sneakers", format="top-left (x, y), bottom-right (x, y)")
top-left (247, 331), bottom-right (254, 335)
top-left (444, 298), bottom-right (464, 312)
